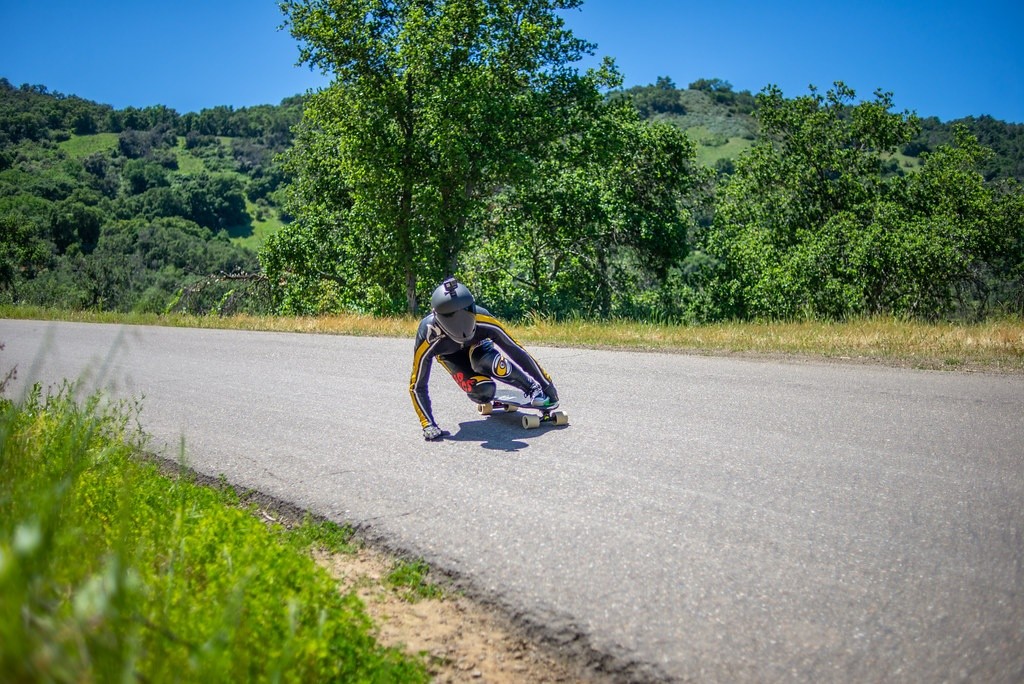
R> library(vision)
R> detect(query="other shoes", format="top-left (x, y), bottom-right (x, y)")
top-left (524, 380), bottom-right (546, 407)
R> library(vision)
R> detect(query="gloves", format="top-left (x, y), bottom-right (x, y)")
top-left (543, 383), bottom-right (559, 403)
top-left (423, 424), bottom-right (442, 441)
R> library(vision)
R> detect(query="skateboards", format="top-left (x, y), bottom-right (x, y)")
top-left (477, 389), bottom-right (569, 430)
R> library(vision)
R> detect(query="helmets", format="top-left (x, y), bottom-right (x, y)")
top-left (431, 277), bottom-right (477, 344)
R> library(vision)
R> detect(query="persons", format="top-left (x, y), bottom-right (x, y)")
top-left (408, 277), bottom-right (569, 439)
